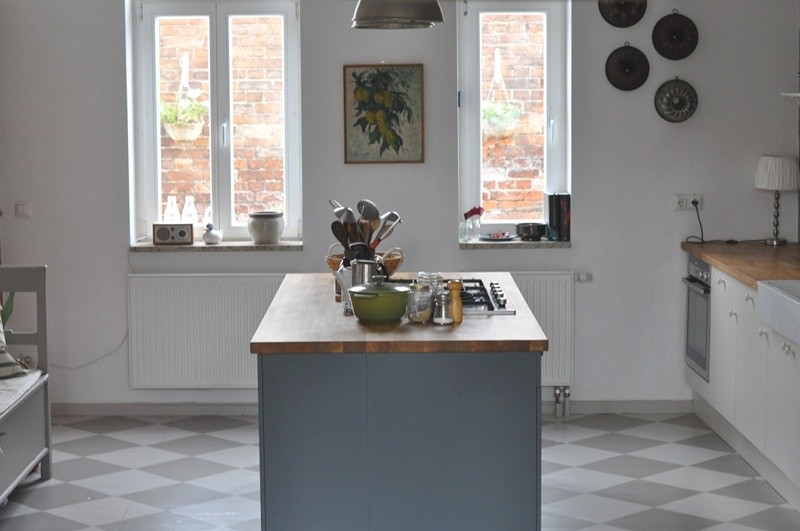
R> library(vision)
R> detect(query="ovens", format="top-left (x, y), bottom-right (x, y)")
top-left (675, 235), bottom-right (723, 385)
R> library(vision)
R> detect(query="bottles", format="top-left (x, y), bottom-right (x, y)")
top-left (459, 212), bottom-right (481, 242)
top-left (406, 270), bottom-right (464, 324)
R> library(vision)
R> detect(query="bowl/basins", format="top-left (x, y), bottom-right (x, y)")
top-left (517, 220), bottom-right (547, 241)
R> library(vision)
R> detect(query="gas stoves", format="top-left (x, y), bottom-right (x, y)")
top-left (377, 272), bottom-right (517, 315)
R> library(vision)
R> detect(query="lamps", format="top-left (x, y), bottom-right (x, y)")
top-left (352, 0), bottom-right (445, 24)
top-left (754, 153), bottom-right (800, 247)
top-left (351, 20), bottom-right (436, 30)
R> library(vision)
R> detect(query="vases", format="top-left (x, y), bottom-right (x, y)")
top-left (248, 209), bottom-right (284, 243)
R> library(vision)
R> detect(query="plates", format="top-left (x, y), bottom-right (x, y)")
top-left (478, 234), bottom-right (515, 239)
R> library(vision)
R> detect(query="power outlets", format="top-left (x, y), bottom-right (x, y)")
top-left (672, 194), bottom-right (688, 211)
top-left (687, 193), bottom-right (702, 211)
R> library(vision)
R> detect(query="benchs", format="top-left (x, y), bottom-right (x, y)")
top-left (0, 264), bottom-right (53, 506)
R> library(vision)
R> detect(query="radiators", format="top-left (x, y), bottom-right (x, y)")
top-left (127, 274), bottom-right (284, 386)
top-left (513, 271), bottom-right (574, 387)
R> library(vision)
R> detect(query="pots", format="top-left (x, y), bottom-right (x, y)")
top-left (347, 274), bottom-right (411, 322)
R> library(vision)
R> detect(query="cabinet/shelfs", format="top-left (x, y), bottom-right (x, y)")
top-left (681, 238), bottom-right (799, 513)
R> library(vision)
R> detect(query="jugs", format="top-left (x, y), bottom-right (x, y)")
top-left (350, 258), bottom-right (389, 287)
